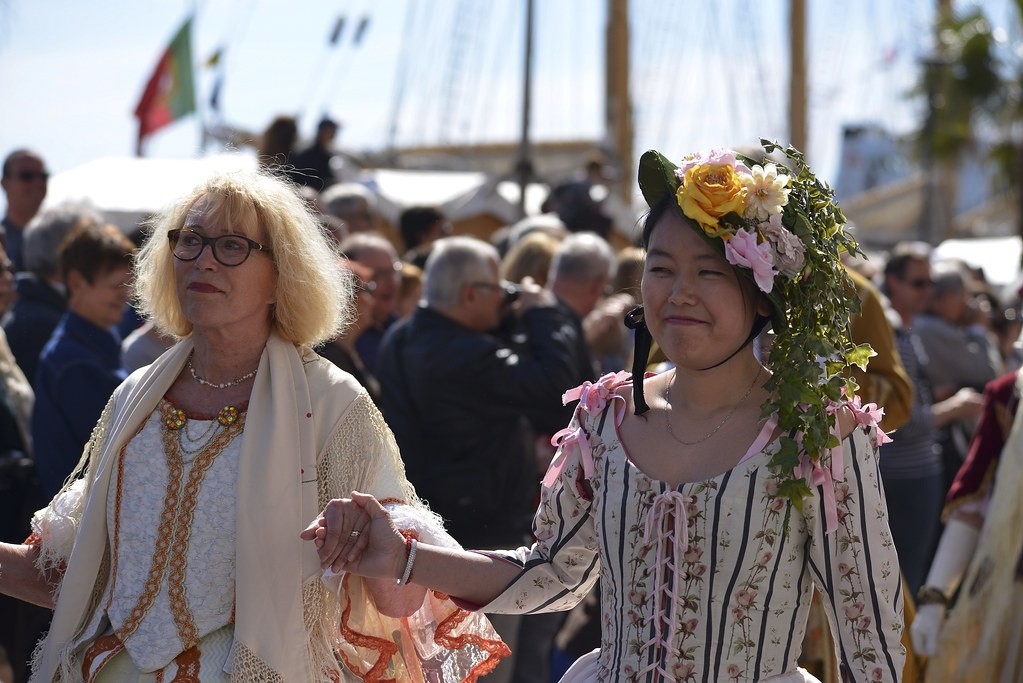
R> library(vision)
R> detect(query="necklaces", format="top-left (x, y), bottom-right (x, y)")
top-left (185, 359), bottom-right (261, 387)
top-left (665, 363), bottom-right (764, 443)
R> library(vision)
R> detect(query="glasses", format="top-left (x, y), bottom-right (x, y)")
top-left (473, 279), bottom-right (517, 297)
top-left (913, 279), bottom-right (923, 288)
top-left (18, 170), bottom-right (49, 182)
top-left (167, 229), bottom-right (270, 266)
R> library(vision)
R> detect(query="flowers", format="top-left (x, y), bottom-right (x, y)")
top-left (677, 134), bottom-right (889, 510)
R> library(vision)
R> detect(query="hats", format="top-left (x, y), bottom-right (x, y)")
top-left (638, 138), bottom-right (790, 334)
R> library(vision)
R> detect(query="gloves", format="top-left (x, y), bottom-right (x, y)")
top-left (909, 520), bottom-right (980, 655)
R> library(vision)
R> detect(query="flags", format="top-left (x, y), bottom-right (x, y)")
top-left (135, 14), bottom-right (202, 158)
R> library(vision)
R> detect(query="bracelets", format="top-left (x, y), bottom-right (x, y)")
top-left (915, 585), bottom-right (951, 609)
top-left (403, 535), bottom-right (416, 586)
top-left (400, 539), bottom-right (416, 590)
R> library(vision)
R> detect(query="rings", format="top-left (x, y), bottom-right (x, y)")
top-left (350, 530), bottom-right (360, 537)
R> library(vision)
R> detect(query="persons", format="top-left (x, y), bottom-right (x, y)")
top-left (1, 118), bottom-right (1023, 683)
